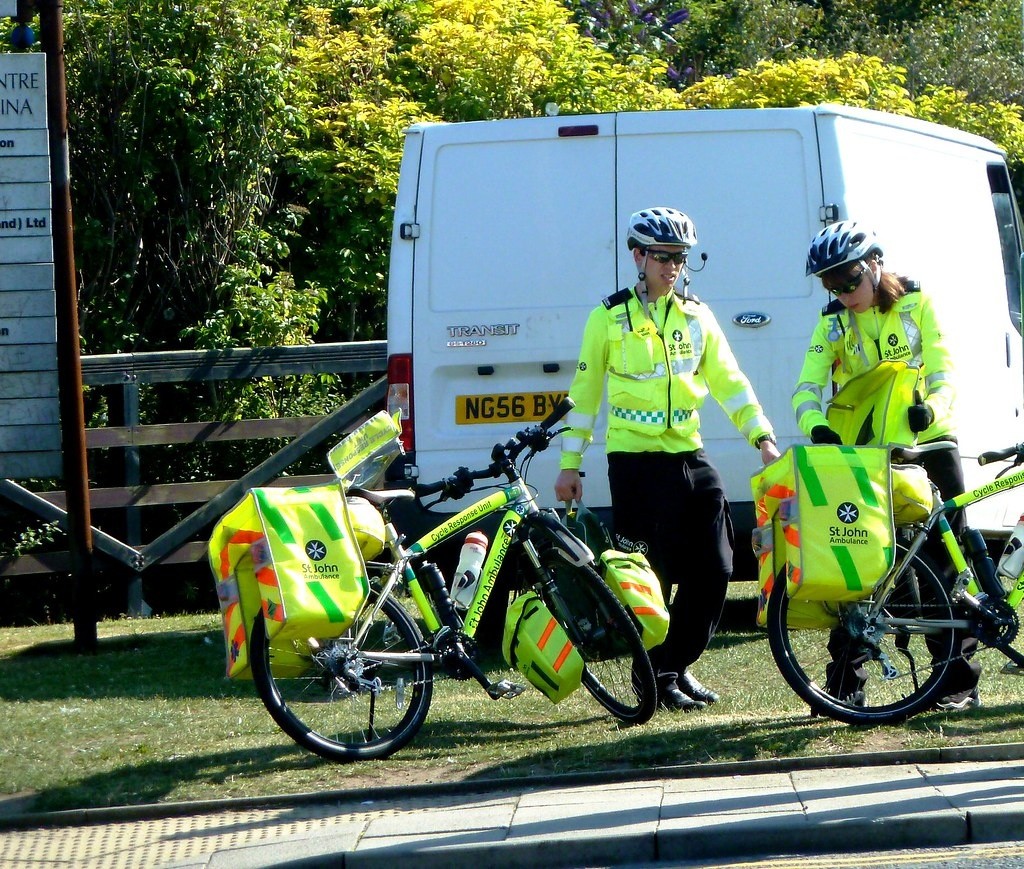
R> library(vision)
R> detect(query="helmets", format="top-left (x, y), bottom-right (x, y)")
top-left (804, 220), bottom-right (884, 278)
top-left (626, 206), bottom-right (697, 257)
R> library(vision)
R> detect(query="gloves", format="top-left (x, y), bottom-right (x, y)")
top-left (811, 425), bottom-right (842, 445)
top-left (907, 404), bottom-right (933, 434)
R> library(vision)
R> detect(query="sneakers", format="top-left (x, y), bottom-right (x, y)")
top-left (677, 672), bottom-right (720, 703)
top-left (935, 685), bottom-right (980, 710)
top-left (638, 683), bottom-right (707, 712)
top-left (811, 687), bottom-right (864, 719)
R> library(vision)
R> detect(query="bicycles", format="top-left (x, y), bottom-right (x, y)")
top-left (239, 395), bottom-right (661, 765)
top-left (763, 442), bottom-right (1022, 728)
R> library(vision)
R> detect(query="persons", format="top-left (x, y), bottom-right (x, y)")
top-left (554, 208), bottom-right (782, 713)
top-left (792, 220), bottom-right (984, 714)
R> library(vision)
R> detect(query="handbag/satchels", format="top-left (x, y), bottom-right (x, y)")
top-left (502, 495), bottom-right (671, 706)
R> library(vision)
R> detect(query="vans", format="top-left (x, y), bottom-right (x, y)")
top-left (386, 104), bottom-right (1023, 574)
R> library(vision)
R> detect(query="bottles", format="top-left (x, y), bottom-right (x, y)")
top-left (418, 559), bottom-right (465, 631)
top-left (999, 511), bottom-right (1023, 580)
top-left (449, 531), bottom-right (488, 610)
top-left (961, 525), bottom-right (1004, 601)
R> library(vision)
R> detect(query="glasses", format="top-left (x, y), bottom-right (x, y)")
top-left (637, 246), bottom-right (689, 266)
top-left (828, 262), bottom-right (871, 297)
